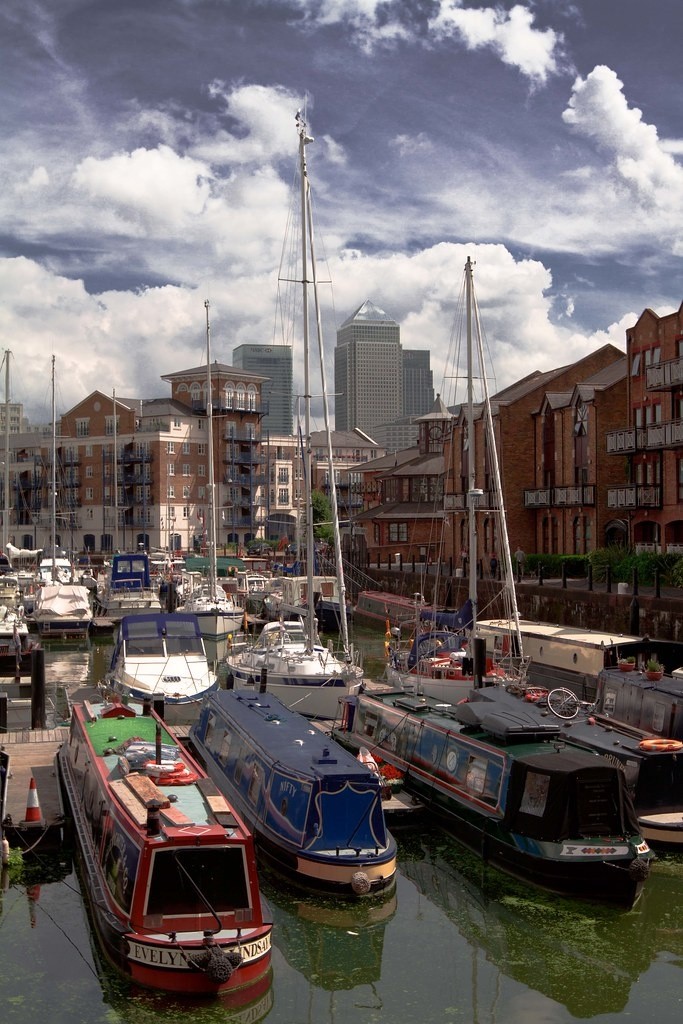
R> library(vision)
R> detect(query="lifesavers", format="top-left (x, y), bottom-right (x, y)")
top-left (525, 692), bottom-right (550, 702)
top-left (228, 565), bottom-right (238, 573)
top-left (639, 738), bottom-right (683, 751)
top-left (143, 759), bottom-right (199, 786)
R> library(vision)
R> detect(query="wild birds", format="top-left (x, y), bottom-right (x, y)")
top-left (536, 621), bottom-right (622, 644)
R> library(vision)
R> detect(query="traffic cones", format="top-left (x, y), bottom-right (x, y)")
top-left (20, 777), bottom-right (46, 827)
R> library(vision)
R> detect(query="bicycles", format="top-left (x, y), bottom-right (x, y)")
top-left (546, 687), bottom-right (596, 720)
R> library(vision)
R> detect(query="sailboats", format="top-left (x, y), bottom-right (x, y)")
top-left (0, 94), bottom-right (683, 856)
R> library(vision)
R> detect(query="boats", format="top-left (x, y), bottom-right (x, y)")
top-left (53, 701), bottom-right (274, 997)
top-left (328, 677), bottom-right (659, 913)
top-left (186, 666), bottom-right (398, 896)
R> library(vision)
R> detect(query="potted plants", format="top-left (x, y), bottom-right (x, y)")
top-left (646, 659), bottom-right (665, 680)
top-left (617, 656), bottom-right (635, 672)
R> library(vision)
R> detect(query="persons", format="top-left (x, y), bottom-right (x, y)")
top-left (514, 545), bottom-right (527, 578)
top-left (314, 539), bottom-right (333, 557)
top-left (457, 544), bottom-right (469, 568)
top-left (159, 580), bottom-right (169, 609)
top-left (489, 555), bottom-right (498, 578)
